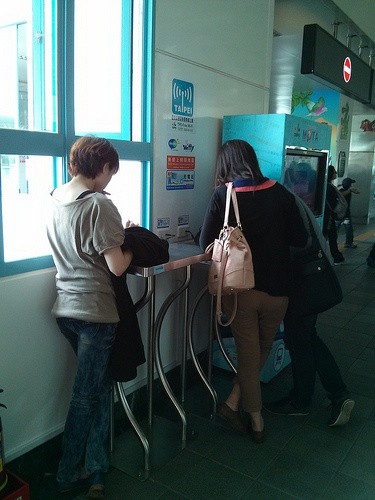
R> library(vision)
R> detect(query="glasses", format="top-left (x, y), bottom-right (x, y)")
top-left (331, 171), bottom-right (336, 174)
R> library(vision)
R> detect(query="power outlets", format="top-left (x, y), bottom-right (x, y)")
top-left (158, 229), bottom-right (170, 238)
top-left (178, 224), bottom-right (190, 237)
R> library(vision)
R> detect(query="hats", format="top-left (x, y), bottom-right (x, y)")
top-left (341, 178), bottom-right (356, 190)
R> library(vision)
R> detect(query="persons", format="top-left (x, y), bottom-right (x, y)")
top-left (199, 140), bottom-right (306, 441)
top-left (366, 243), bottom-right (375, 265)
top-left (267, 177), bottom-right (356, 429)
top-left (338, 178), bottom-right (360, 248)
top-left (323, 165), bottom-right (348, 265)
top-left (46, 136), bottom-right (136, 500)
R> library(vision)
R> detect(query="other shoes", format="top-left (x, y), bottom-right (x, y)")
top-left (334, 258), bottom-right (345, 266)
top-left (328, 397), bottom-right (356, 426)
top-left (263, 398), bottom-right (311, 415)
top-left (248, 416), bottom-right (265, 436)
top-left (56, 478), bottom-right (90, 493)
top-left (216, 402), bottom-right (245, 432)
top-left (85, 470), bottom-right (105, 500)
top-left (344, 243), bottom-right (357, 249)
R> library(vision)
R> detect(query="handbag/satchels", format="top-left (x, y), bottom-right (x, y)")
top-left (331, 199), bottom-right (348, 220)
top-left (204, 181), bottom-right (255, 296)
top-left (290, 253), bottom-right (344, 318)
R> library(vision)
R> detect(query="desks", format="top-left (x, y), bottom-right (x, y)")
top-left (110, 238), bottom-right (242, 482)
